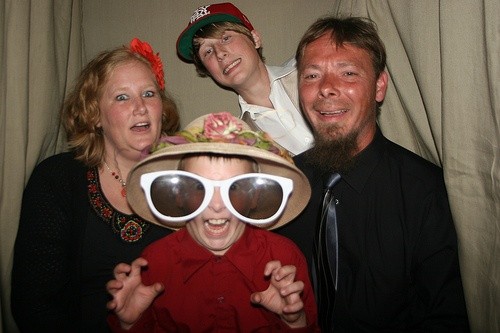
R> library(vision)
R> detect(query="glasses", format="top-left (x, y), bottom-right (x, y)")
top-left (139, 169), bottom-right (295, 224)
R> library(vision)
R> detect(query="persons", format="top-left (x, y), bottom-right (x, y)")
top-left (8, 39), bottom-right (181, 333)
top-left (104, 108), bottom-right (318, 333)
top-left (174, 2), bottom-right (317, 158)
top-left (240, 15), bottom-right (471, 333)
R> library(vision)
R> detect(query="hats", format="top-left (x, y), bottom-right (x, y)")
top-left (176, 2), bottom-right (253, 61)
top-left (123, 113), bottom-right (311, 230)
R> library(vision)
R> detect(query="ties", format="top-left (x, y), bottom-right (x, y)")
top-left (316, 173), bottom-right (341, 325)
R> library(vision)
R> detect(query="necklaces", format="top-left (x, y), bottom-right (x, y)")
top-left (99, 146), bottom-right (132, 196)
